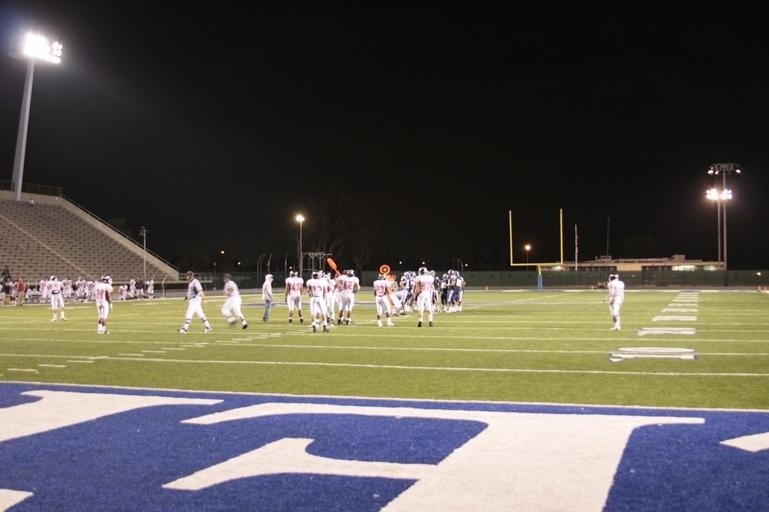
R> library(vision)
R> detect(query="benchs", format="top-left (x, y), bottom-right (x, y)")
top-left (0, 201), bottom-right (173, 285)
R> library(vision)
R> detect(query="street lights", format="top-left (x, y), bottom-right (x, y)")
top-left (5, 27), bottom-right (69, 196)
top-left (707, 161), bottom-right (742, 263)
top-left (296, 212), bottom-right (307, 278)
top-left (703, 186), bottom-right (735, 262)
top-left (524, 243), bottom-right (532, 271)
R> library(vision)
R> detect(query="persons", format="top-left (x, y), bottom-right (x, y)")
top-left (29, 197), bottom-right (34, 206)
top-left (607, 274), bottom-right (625, 331)
